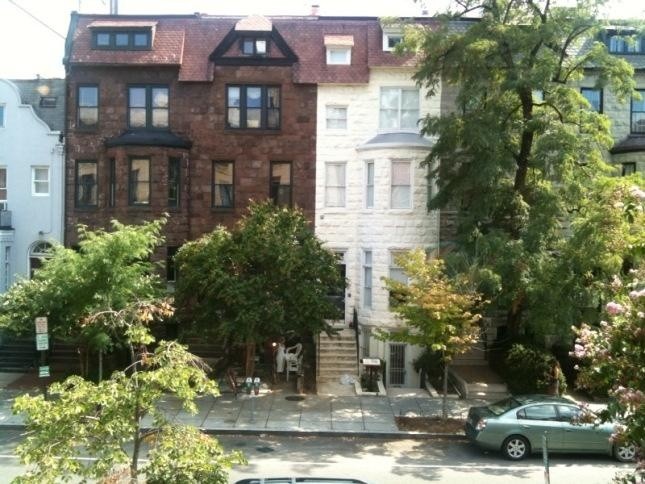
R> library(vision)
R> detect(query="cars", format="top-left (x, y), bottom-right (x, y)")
top-left (463, 390), bottom-right (639, 463)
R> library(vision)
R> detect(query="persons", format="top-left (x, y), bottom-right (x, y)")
top-left (274, 337), bottom-right (286, 381)
top-left (608, 385), bottom-right (618, 409)
top-left (554, 362), bottom-right (560, 394)
top-left (297, 358), bottom-right (310, 393)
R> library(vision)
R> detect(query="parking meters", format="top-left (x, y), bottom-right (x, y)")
top-left (245, 376), bottom-right (261, 423)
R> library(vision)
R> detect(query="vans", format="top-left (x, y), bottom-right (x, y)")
top-left (238, 472), bottom-right (368, 483)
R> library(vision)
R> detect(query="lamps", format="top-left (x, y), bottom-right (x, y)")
top-left (37, 78), bottom-right (48, 97)
top-left (57, 132), bottom-right (66, 143)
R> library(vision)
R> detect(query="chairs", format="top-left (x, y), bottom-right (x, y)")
top-left (286, 343), bottom-right (302, 382)
top-left (227, 368), bottom-right (247, 400)
